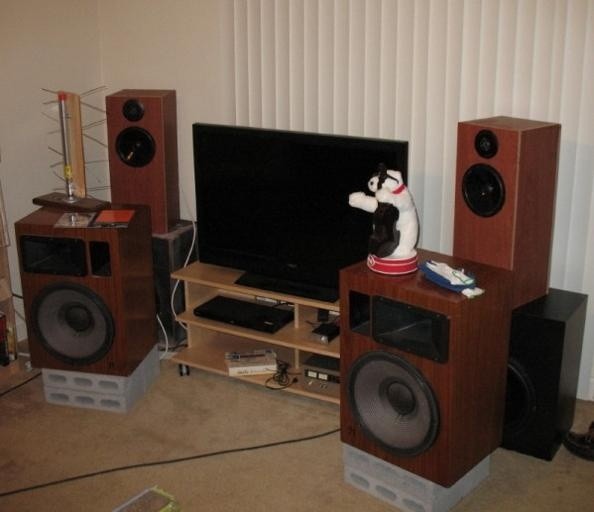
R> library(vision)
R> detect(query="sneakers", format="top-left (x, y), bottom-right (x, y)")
top-left (562, 427), bottom-right (594, 463)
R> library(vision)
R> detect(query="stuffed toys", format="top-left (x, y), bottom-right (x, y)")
top-left (348, 169), bottom-right (419, 275)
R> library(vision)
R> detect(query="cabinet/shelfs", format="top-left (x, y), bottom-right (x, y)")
top-left (164, 259), bottom-right (346, 408)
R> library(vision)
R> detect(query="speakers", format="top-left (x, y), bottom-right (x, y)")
top-left (105, 87), bottom-right (179, 234)
top-left (339, 249), bottom-right (511, 489)
top-left (452, 114), bottom-right (560, 307)
top-left (13, 202), bottom-right (157, 376)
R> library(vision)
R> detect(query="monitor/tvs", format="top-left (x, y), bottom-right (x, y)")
top-left (192, 122), bottom-right (408, 304)
